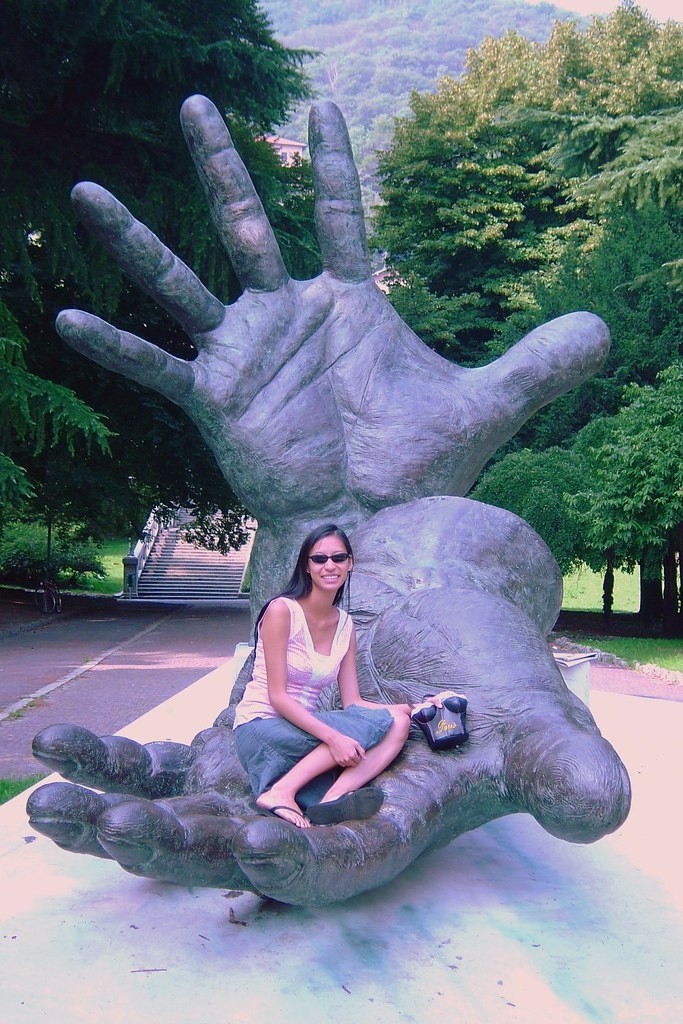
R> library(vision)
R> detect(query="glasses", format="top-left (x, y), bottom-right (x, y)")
top-left (308, 553), bottom-right (349, 563)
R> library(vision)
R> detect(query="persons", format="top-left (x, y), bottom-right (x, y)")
top-left (57, 92), bottom-right (612, 643)
top-left (232, 523), bottom-right (468, 829)
top-left (26, 495), bottom-right (631, 904)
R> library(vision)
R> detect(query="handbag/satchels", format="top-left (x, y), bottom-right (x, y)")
top-left (408, 690), bottom-right (469, 751)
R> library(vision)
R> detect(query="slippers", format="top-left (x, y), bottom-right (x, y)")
top-left (306, 787), bottom-right (383, 825)
top-left (256, 802), bottom-right (315, 828)
